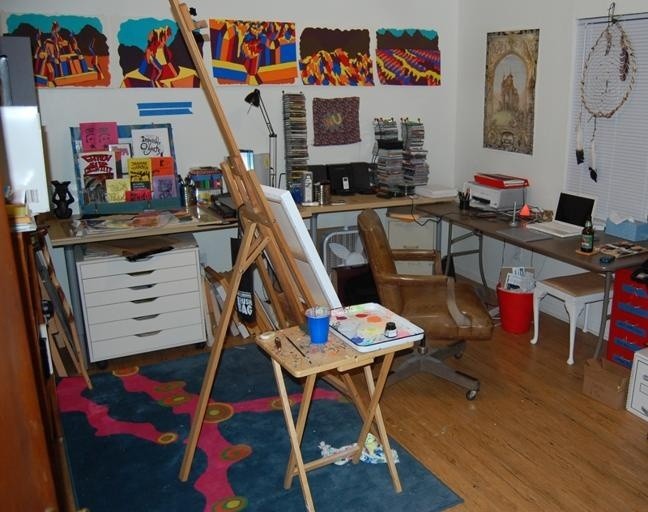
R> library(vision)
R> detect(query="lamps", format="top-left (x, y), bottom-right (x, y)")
top-left (243, 87), bottom-right (282, 190)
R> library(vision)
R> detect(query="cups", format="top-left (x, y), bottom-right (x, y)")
top-left (459, 198), bottom-right (472, 213)
top-left (305, 306), bottom-right (332, 344)
top-left (313, 179), bottom-right (331, 206)
top-left (181, 184), bottom-right (196, 207)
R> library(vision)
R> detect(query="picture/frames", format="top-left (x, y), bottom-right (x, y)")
top-left (255, 184), bottom-right (341, 309)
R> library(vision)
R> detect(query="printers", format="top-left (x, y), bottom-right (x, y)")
top-left (463, 179), bottom-right (524, 212)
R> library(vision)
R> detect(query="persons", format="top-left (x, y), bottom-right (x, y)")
top-left (52, 188), bottom-right (74, 219)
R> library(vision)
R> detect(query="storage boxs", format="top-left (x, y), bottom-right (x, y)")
top-left (580, 358), bottom-right (628, 411)
top-left (624, 346), bottom-right (647, 423)
top-left (464, 180), bottom-right (532, 213)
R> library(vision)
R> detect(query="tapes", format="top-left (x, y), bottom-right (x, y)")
top-left (260, 331), bottom-right (275, 340)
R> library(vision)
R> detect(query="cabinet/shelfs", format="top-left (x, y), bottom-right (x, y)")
top-left (605, 267), bottom-right (647, 368)
top-left (0, 177), bottom-right (65, 511)
top-left (73, 231), bottom-right (207, 366)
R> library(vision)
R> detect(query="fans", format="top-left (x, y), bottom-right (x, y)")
top-left (319, 228), bottom-right (370, 293)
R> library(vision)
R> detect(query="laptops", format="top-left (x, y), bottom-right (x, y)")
top-left (526, 190), bottom-right (597, 238)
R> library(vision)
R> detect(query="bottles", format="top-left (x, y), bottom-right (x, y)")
top-left (580, 214), bottom-right (595, 253)
top-left (385, 321), bottom-right (398, 338)
top-left (303, 175), bottom-right (314, 201)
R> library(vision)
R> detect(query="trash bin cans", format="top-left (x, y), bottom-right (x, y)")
top-left (497, 282), bottom-right (534, 335)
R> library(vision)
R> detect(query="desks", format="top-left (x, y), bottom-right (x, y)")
top-left (303, 182), bottom-right (462, 309)
top-left (254, 318), bottom-right (425, 512)
top-left (417, 194), bottom-right (647, 367)
top-left (37, 202), bottom-right (312, 374)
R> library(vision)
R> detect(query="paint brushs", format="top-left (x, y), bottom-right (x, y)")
top-left (283, 332), bottom-right (306, 358)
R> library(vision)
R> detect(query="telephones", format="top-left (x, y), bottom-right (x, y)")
top-left (335, 177), bottom-right (359, 196)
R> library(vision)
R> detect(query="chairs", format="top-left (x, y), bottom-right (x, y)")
top-left (350, 202), bottom-right (494, 399)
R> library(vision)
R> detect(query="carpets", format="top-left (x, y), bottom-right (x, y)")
top-left (50, 336), bottom-right (469, 512)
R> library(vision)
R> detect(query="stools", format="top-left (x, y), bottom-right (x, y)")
top-left (528, 273), bottom-right (619, 367)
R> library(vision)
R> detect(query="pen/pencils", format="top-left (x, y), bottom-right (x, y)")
top-left (458, 187), bottom-right (470, 201)
top-left (179, 174), bottom-right (192, 186)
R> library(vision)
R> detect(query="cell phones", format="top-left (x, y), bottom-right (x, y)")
top-left (601, 255), bottom-right (615, 263)
